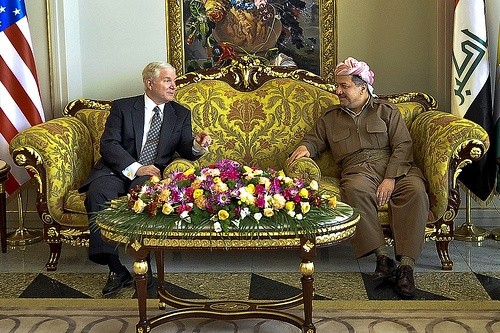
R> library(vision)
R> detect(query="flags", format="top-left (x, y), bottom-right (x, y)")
top-left (0, 0), bottom-right (46, 199)
top-left (450, 0), bottom-right (500, 209)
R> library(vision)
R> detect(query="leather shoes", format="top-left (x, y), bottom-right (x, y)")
top-left (102, 264), bottom-right (133, 296)
top-left (134, 256), bottom-right (153, 288)
top-left (396, 262), bottom-right (416, 296)
top-left (372, 254), bottom-right (397, 281)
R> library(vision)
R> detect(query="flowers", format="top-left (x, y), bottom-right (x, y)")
top-left (124, 162), bottom-right (327, 225)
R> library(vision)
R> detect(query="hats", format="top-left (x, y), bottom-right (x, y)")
top-left (335, 56), bottom-right (378, 99)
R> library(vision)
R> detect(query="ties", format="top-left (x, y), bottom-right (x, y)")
top-left (137, 106), bottom-right (162, 165)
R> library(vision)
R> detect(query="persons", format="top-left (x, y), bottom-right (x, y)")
top-left (77, 61), bottom-right (213, 296)
top-left (285, 55), bottom-right (431, 298)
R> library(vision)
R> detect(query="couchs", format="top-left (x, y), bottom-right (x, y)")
top-left (8, 55), bottom-right (489, 273)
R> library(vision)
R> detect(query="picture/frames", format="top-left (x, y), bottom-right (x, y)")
top-left (166, 0), bottom-right (339, 92)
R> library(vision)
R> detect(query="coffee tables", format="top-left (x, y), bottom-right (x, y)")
top-left (94, 203), bottom-right (361, 332)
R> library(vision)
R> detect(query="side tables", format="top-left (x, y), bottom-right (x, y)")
top-left (0, 160), bottom-right (10, 253)
